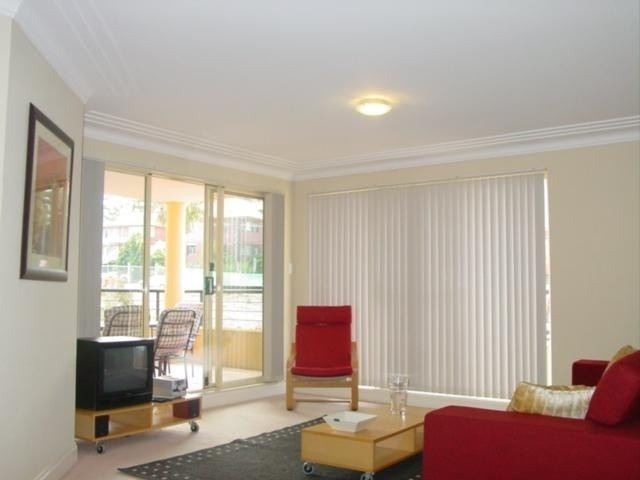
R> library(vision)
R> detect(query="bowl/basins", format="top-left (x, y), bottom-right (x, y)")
top-left (323, 410), bottom-right (378, 434)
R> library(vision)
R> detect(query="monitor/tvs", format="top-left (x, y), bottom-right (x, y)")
top-left (75, 335), bottom-right (155, 412)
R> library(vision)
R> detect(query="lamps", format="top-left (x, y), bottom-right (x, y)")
top-left (351, 95), bottom-right (394, 118)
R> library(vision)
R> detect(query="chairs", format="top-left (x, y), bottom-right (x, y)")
top-left (102, 302), bottom-right (148, 337)
top-left (284, 303), bottom-right (361, 414)
top-left (148, 307), bottom-right (195, 390)
top-left (173, 300), bottom-right (206, 380)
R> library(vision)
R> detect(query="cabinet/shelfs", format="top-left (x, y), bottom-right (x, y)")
top-left (74, 395), bottom-right (204, 456)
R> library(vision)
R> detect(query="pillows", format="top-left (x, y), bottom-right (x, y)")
top-left (505, 380), bottom-right (596, 422)
top-left (586, 349), bottom-right (640, 428)
top-left (599, 343), bottom-right (633, 363)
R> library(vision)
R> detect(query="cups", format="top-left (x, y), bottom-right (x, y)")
top-left (387, 373), bottom-right (410, 416)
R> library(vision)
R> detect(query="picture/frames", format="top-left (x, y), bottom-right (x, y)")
top-left (18, 101), bottom-right (76, 283)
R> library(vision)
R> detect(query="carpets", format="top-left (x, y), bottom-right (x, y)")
top-left (116, 409), bottom-right (424, 480)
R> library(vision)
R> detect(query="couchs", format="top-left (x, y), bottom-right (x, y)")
top-left (423, 343), bottom-right (640, 480)
